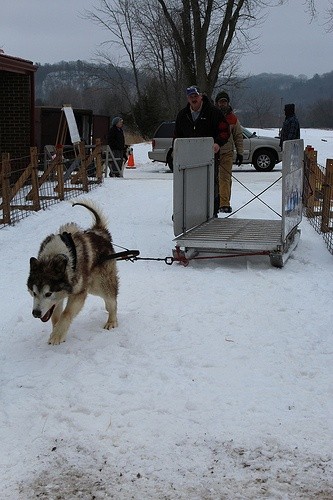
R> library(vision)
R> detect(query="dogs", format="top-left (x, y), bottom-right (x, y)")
top-left (26, 198), bottom-right (121, 346)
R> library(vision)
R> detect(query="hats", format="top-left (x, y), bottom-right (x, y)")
top-left (283, 104), bottom-right (294, 111)
top-left (214, 91), bottom-right (229, 103)
top-left (186, 86), bottom-right (203, 97)
top-left (111, 117), bottom-right (122, 126)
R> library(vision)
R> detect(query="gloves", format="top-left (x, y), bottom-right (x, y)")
top-left (235, 154), bottom-right (243, 167)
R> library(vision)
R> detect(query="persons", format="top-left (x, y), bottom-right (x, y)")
top-left (172, 86), bottom-right (244, 220)
top-left (279, 104), bottom-right (300, 151)
top-left (108, 117), bottom-right (126, 177)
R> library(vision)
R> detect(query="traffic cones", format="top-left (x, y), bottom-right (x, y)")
top-left (125, 150), bottom-right (137, 169)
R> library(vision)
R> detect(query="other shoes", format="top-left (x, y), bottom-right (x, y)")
top-left (220, 206), bottom-right (231, 213)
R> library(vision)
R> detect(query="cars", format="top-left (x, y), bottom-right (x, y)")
top-left (150, 121), bottom-right (283, 174)
